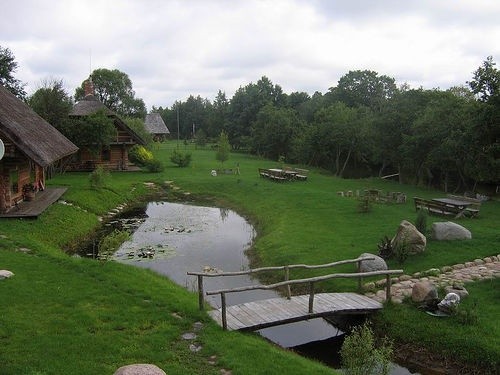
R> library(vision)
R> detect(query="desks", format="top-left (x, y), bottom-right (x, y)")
top-left (432, 198), bottom-right (481, 221)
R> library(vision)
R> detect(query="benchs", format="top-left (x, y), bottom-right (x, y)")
top-left (259, 166), bottom-right (310, 183)
top-left (445, 205), bottom-right (481, 221)
top-left (413, 196), bottom-right (454, 217)
